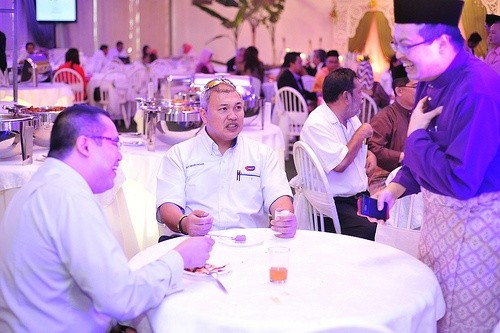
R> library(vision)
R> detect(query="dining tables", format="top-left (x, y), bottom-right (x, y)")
top-left (128, 227), bottom-right (438, 333)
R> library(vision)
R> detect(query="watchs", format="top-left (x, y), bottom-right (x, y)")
top-left (176, 214), bottom-right (188, 235)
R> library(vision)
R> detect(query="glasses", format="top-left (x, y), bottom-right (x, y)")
top-left (202, 78), bottom-right (236, 93)
top-left (85, 135), bottom-right (122, 148)
top-left (389, 40), bottom-right (435, 53)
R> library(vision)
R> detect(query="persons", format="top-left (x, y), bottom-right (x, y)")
top-left (0, 104), bottom-right (214, 331)
top-left (305, 48), bottom-right (327, 75)
top-left (155, 77), bottom-right (298, 244)
top-left (357, 0), bottom-right (500, 332)
top-left (91, 34), bottom-right (269, 85)
top-left (53, 47), bottom-right (89, 102)
top-left (363, 63), bottom-right (420, 172)
top-left (483, 19), bottom-right (500, 74)
top-left (381, 52), bottom-right (403, 100)
top-left (484, 12), bottom-right (500, 53)
top-left (312, 49), bottom-right (342, 93)
top-left (276, 51), bottom-right (322, 112)
top-left (301, 68), bottom-right (378, 241)
top-left (463, 30), bottom-right (483, 56)
top-left (17, 41), bottom-right (36, 66)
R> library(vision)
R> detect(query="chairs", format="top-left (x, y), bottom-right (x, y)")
top-left (0, 49), bottom-right (422, 235)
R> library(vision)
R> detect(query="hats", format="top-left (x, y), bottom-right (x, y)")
top-left (392, 64), bottom-right (407, 80)
top-left (393, 0), bottom-right (464, 26)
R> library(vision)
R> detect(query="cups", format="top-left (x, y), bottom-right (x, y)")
top-left (268, 245), bottom-right (290, 284)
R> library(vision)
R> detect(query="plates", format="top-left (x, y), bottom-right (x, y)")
top-left (183, 253), bottom-right (235, 277)
top-left (218, 233), bottom-right (264, 247)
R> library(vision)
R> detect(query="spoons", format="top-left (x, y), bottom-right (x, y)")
top-left (207, 272), bottom-right (228, 293)
